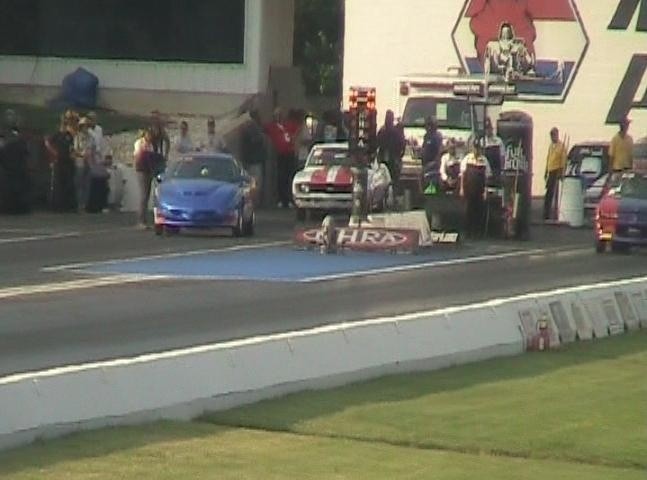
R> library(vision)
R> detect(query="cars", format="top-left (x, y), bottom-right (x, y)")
top-left (590, 166), bottom-right (646, 255)
top-left (152, 150), bottom-right (258, 240)
top-left (290, 139), bottom-right (391, 223)
top-left (630, 138), bottom-right (646, 179)
top-left (555, 143), bottom-right (610, 212)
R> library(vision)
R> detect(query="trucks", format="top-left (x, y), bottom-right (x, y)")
top-left (392, 78), bottom-right (535, 224)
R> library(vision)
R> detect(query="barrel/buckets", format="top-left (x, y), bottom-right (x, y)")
top-left (556, 177), bottom-right (586, 227)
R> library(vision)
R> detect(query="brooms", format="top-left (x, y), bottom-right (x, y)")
top-left (542, 136), bottom-right (574, 226)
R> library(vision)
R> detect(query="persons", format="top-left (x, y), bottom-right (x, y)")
top-left (607, 118), bottom-right (634, 187)
top-left (364, 153), bottom-right (394, 215)
top-left (542, 128), bottom-right (568, 225)
top-left (1, 108), bottom-right (113, 215)
top-left (375, 111), bottom-right (406, 212)
top-left (238, 105), bottom-right (350, 211)
top-left (557, 54), bottom-right (565, 87)
top-left (133, 111), bottom-right (171, 232)
top-left (196, 116), bottom-right (228, 154)
top-left (175, 121), bottom-right (194, 154)
top-left (420, 116), bottom-right (506, 238)
